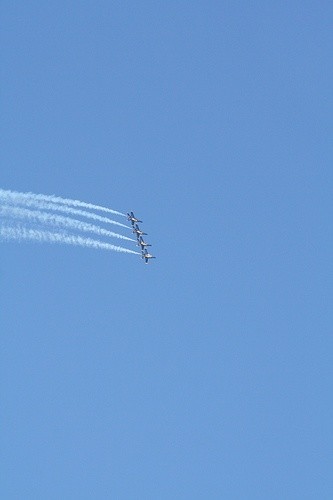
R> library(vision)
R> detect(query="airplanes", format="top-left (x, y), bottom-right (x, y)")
top-left (128, 212), bottom-right (156, 264)
